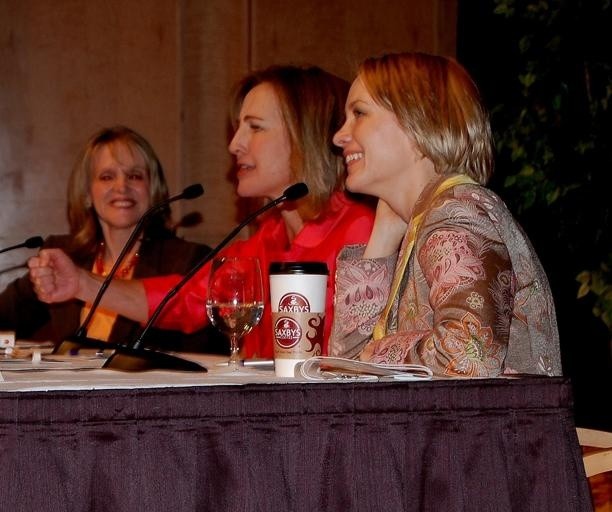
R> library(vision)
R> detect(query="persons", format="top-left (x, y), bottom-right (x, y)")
top-left (25, 61), bottom-right (375, 362)
top-left (0, 125), bottom-right (236, 358)
top-left (326, 49), bottom-right (562, 380)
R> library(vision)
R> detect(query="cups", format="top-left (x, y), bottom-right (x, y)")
top-left (268, 259), bottom-right (329, 378)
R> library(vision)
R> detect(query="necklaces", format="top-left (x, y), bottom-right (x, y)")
top-left (93, 244), bottom-right (139, 279)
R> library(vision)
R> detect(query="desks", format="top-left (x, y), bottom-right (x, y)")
top-left (0, 353), bottom-right (595, 512)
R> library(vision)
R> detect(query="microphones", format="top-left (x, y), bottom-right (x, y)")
top-left (101, 182), bottom-right (309, 373)
top-left (51, 184), bottom-right (205, 355)
top-left (0, 236), bottom-right (44, 253)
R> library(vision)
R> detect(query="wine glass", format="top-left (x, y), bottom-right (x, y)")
top-left (206, 256), bottom-right (264, 374)
